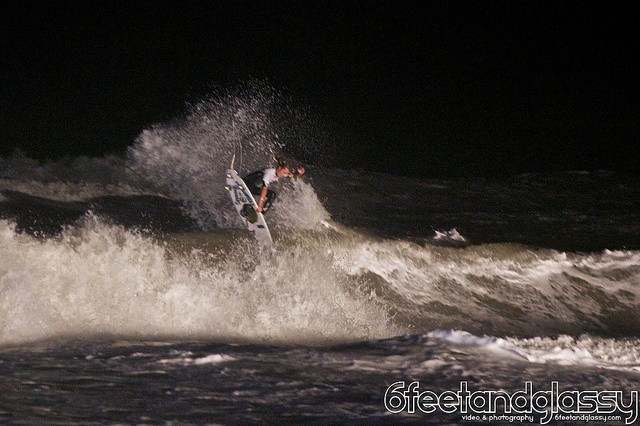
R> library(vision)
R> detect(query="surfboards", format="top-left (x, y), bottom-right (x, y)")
top-left (225, 169), bottom-right (274, 249)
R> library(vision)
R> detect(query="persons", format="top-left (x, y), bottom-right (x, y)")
top-left (289, 166), bottom-right (306, 178)
top-left (243, 164), bottom-right (290, 215)
